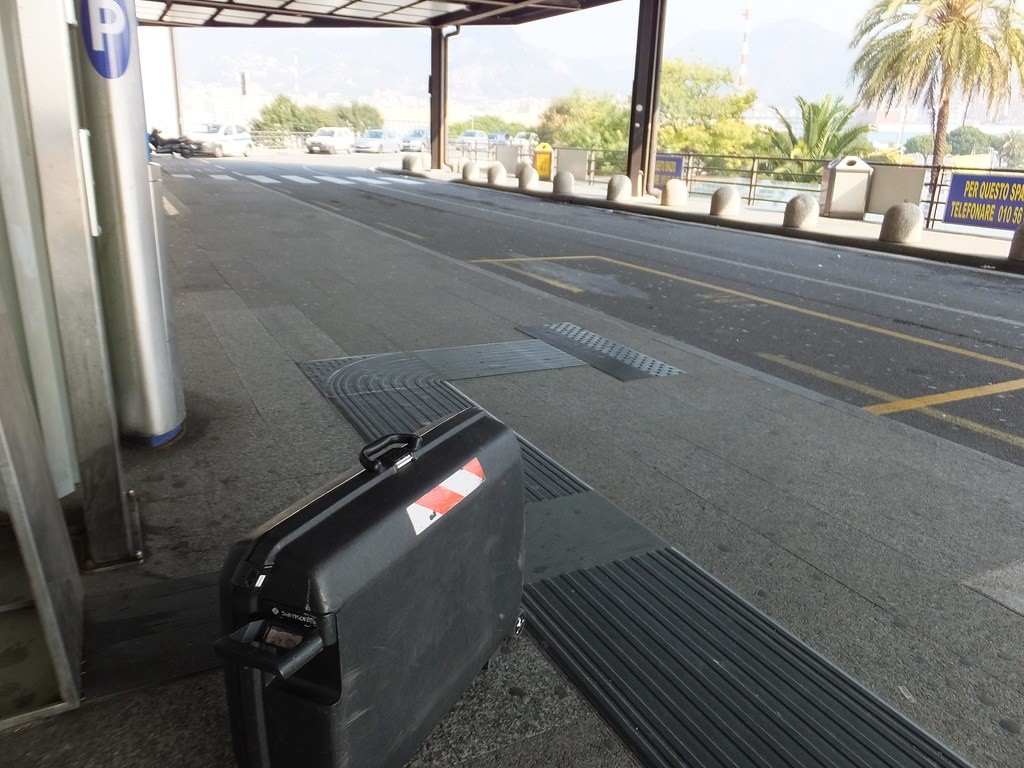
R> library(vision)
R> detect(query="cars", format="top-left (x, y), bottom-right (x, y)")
top-left (357, 129), bottom-right (404, 153)
top-left (186, 123), bottom-right (252, 158)
top-left (401, 127), bottom-right (432, 152)
top-left (455, 130), bottom-right (541, 150)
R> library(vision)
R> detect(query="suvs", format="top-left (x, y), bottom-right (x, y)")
top-left (307, 125), bottom-right (360, 154)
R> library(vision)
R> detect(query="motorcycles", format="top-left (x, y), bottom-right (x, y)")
top-left (149, 128), bottom-right (202, 159)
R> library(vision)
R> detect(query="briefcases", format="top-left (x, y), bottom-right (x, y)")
top-left (212, 404), bottom-right (529, 768)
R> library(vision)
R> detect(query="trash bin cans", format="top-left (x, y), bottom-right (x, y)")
top-left (533, 142), bottom-right (553, 181)
top-left (819, 156), bottom-right (875, 220)
